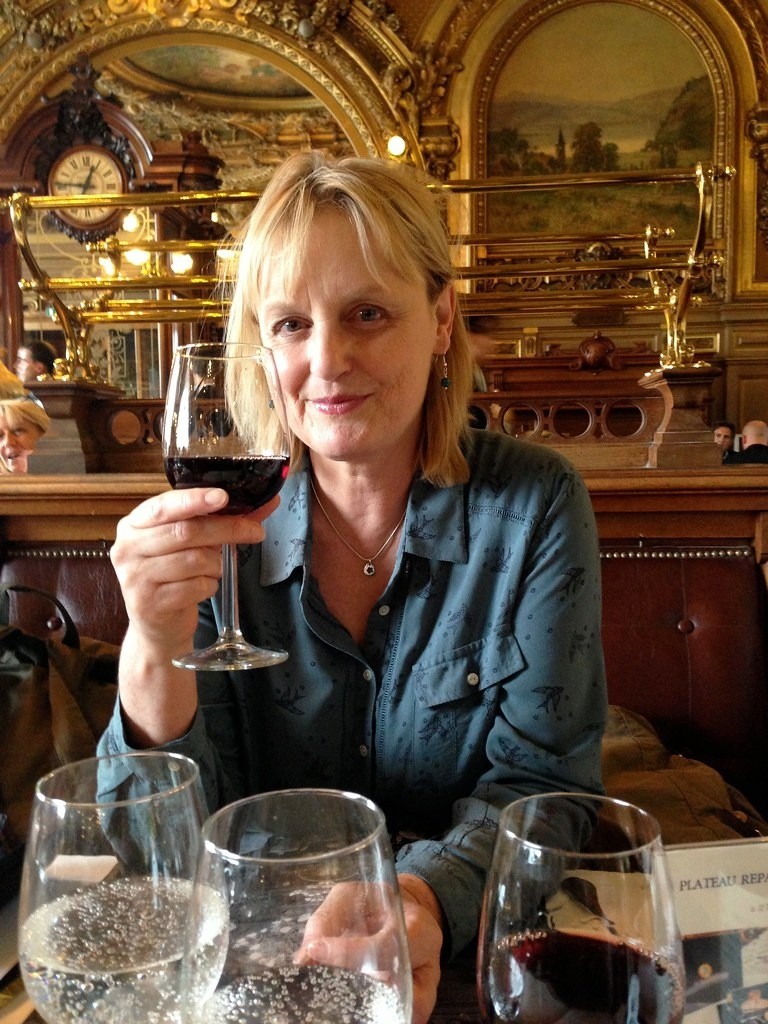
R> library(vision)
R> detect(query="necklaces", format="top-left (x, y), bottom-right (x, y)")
top-left (309, 472), bottom-right (407, 575)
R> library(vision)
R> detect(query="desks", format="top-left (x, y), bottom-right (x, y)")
top-left (0, 893), bottom-right (485, 1024)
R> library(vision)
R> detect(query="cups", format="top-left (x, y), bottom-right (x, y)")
top-left (16, 751), bottom-right (229, 1024)
top-left (474, 791), bottom-right (686, 1023)
top-left (180, 787), bottom-right (413, 1024)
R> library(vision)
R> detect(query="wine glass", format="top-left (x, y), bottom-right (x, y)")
top-left (158, 343), bottom-right (295, 672)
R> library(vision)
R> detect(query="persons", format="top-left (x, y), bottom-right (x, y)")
top-left (95, 148), bottom-right (609, 1024)
top-left (712, 419), bottom-right (768, 465)
top-left (0, 341), bottom-right (58, 475)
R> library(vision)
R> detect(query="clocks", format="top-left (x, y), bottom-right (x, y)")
top-left (32, 52), bottom-right (135, 246)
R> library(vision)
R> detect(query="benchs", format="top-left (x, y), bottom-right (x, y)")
top-left (0, 473), bottom-right (768, 778)
